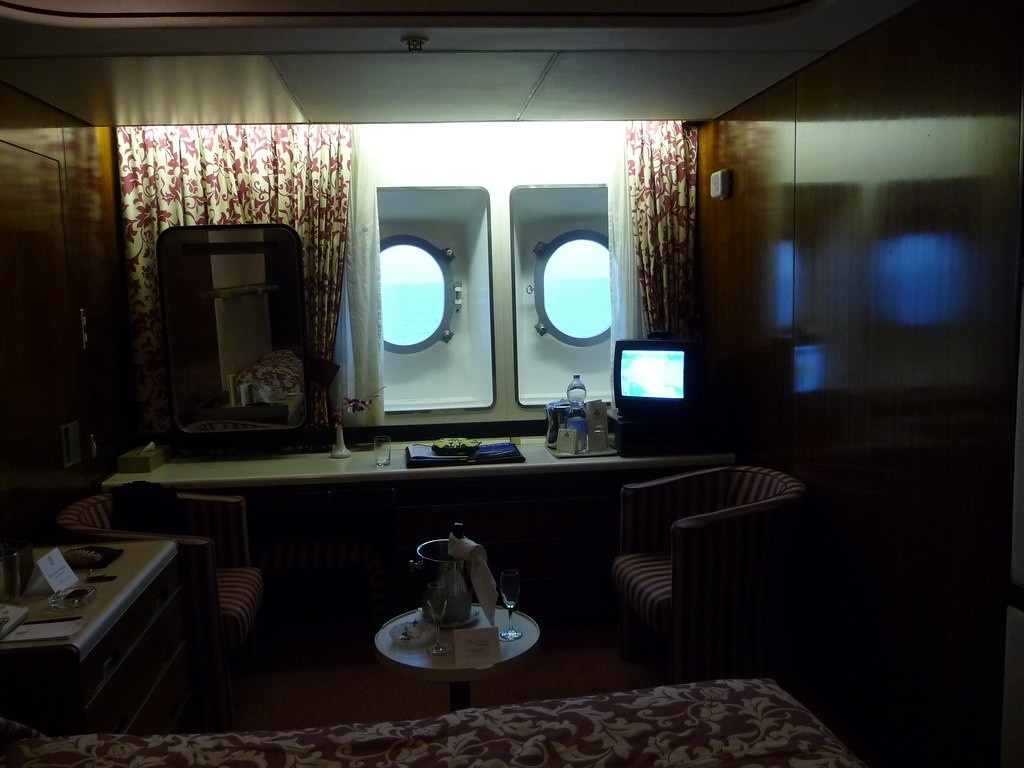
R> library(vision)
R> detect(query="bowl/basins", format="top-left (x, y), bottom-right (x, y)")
top-left (390, 621), bottom-right (434, 647)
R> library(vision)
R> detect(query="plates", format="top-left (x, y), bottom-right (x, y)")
top-left (416, 606), bottom-right (480, 626)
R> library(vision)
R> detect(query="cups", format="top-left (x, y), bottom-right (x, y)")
top-left (3, 548), bottom-right (32, 598)
top-left (374, 436), bottom-right (391, 466)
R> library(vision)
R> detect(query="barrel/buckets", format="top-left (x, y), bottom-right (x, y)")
top-left (409, 539), bottom-right (472, 625)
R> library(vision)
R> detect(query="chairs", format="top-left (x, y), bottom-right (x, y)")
top-left (53, 489), bottom-right (271, 666)
top-left (609, 465), bottom-right (809, 683)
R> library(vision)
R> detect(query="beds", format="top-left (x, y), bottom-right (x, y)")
top-left (0, 677), bottom-right (853, 768)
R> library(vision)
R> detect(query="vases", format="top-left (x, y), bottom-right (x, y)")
top-left (330, 424), bottom-right (352, 458)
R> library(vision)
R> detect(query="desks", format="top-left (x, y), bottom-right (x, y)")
top-left (374, 603), bottom-right (542, 714)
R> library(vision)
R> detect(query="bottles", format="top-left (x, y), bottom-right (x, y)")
top-left (566, 374), bottom-right (588, 453)
top-left (591, 425), bottom-right (603, 453)
top-left (443, 522), bottom-right (464, 561)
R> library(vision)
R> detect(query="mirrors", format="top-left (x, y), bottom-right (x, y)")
top-left (155, 224), bottom-right (311, 438)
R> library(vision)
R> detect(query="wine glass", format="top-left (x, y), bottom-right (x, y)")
top-left (500, 570), bottom-right (523, 640)
top-left (427, 582), bottom-right (453, 656)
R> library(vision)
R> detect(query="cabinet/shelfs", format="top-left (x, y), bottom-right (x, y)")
top-left (0, 556), bottom-right (203, 735)
top-left (0, 79), bottom-right (130, 543)
top-left (244, 473), bottom-right (632, 634)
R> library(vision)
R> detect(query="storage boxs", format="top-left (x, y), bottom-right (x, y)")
top-left (192, 390), bottom-right (304, 423)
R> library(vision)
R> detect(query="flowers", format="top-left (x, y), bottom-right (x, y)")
top-left (325, 385), bottom-right (386, 427)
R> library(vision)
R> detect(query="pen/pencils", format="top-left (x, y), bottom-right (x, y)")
top-left (23, 616), bottom-right (82, 625)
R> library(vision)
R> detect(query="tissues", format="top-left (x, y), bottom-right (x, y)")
top-left (117, 440), bottom-right (172, 473)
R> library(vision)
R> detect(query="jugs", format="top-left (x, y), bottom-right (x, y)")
top-left (544, 401), bottom-right (570, 449)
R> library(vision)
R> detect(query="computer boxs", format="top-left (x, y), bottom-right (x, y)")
top-left (606, 410), bottom-right (701, 457)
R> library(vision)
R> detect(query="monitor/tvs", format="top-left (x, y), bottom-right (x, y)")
top-left (613, 339), bottom-right (701, 418)
